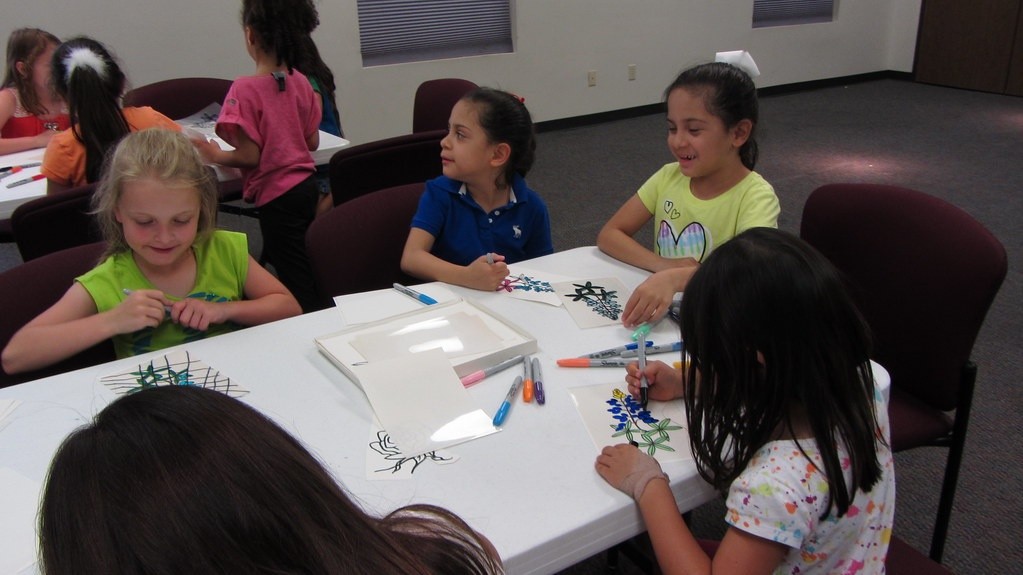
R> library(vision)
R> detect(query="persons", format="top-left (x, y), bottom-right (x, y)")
top-left (42, 35), bottom-right (182, 195)
top-left (595, 62), bottom-right (783, 327)
top-left (594, 226), bottom-right (898, 575)
top-left (184, 0), bottom-right (347, 284)
top-left (397, 86), bottom-right (557, 290)
top-left (0, 125), bottom-right (303, 379)
top-left (40, 382), bottom-right (504, 575)
top-left (0, 27), bottom-right (80, 156)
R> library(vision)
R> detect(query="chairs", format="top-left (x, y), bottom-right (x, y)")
top-left (12, 180), bottom-right (105, 262)
top-left (1, 238), bottom-right (121, 389)
top-left (797, 184), bottom-right (1009, 555)
top-left (413, 78), bottom-right (480, 131)
top-left (305, 175), bottom-right (431, 308)
top-left (331, 129), bottom-right (450, 209)
top-left (123, 77), bottom-right (236, 120)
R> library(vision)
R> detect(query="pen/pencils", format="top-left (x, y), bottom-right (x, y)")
top-left (487, 253), bottom-right (493, 263)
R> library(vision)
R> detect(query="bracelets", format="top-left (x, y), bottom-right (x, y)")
top-left (632, 472), bottom-right (672, 504)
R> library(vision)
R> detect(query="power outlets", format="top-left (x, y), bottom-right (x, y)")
top-left (588, 71), bottom-right (595, 86)
top-left (628, 64), bottom-right (636, 80)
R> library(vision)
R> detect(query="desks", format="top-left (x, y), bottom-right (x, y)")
top-left (1, 245), bottom-right (748, 574)
top-left (0, 129), bottom-right (350, 221)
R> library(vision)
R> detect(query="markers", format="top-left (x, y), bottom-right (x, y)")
top-left (632, 310), bottom-right (669, 340)
top-left (638, 333), bottom-right (648, 413)
top-left (558, 342), bottom-right (685, 368)
top-left (393, 282), bottom-right (438, 307)
top-left (460, 355), bottom-right (544, 425)
top-left (0, 162), bottom-right (43, 178)
top-left (122, 288), bottom-right (172, 312)
top-left (6, 173), bottom-right (46, 188)
